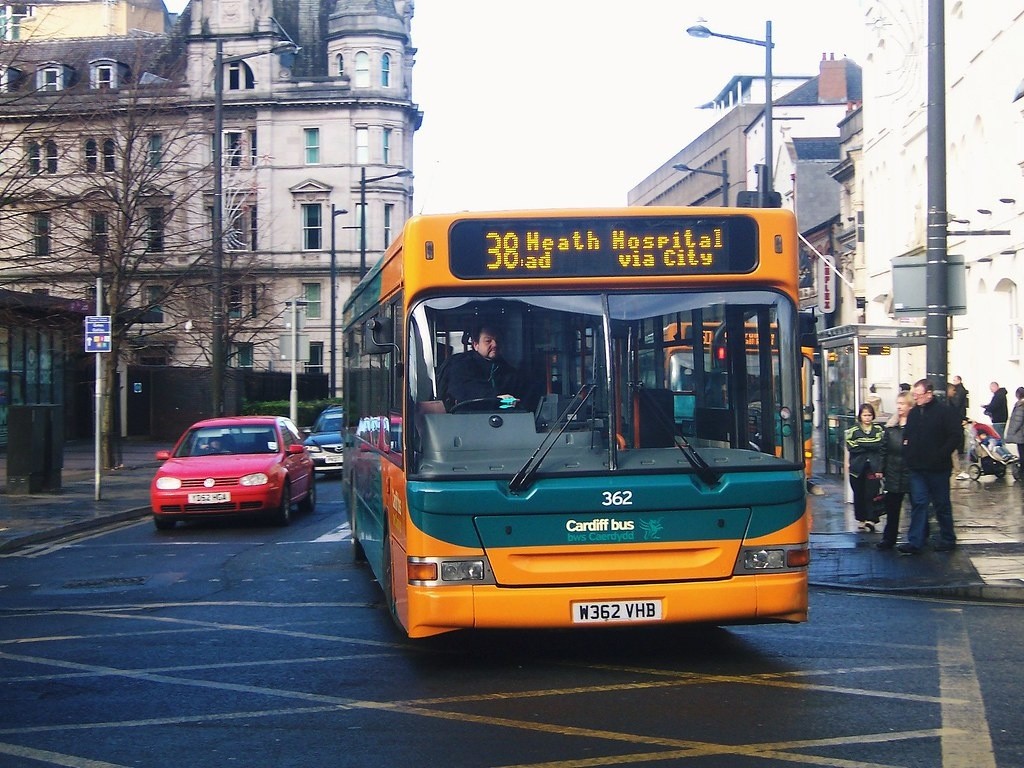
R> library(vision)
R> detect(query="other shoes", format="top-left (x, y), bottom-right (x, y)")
top-left (897, 543), bottom-right (921, 555)
top-left (934, 541), bottom-right (955, 551)
top-left (877, 540), bottom-right (895, 547)
top-left (956, 473), bottom-right (969, 480)
top-left (856, 522), bottom-right (875, 533)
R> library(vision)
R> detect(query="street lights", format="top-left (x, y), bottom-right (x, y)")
top-left (671, 158), bottom-right (729, 209)
top-left (684, 19), bottom-right (777, 208)
top-left (328, 202), bottom-right (348, 407)
top-left (358, 163), bottom-right (412, 285)
top-left (210, 36), bottom-right (297, 419)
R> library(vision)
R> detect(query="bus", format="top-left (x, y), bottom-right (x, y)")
top-left (606, 319), bottom-right (825, 482)
top-left (337, 203), bottom-right (821, 643)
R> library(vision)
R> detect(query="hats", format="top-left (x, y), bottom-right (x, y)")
top-left (978, 429), bottom-right (987, 435)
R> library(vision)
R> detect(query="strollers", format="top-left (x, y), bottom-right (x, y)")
top-left (967, 421), bottom-right (1022, 481)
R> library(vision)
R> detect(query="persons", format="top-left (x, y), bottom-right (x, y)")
top-left (436, 315), bottom-right (528, 411)
top-left (947, 375), bottom-right (966, 454)
top-left (200, 437), bottom-right (230, 452)
top-left (897, 379), bottom-right (961, 553)
top-left (845, 403), bottom-right (885, 531)
top-left (875, 391), bottom-right (930, 548)
top-left (1006, 387), bottom-right (1024, 471)
top-left (980, 381), bottom-right (1008, 437)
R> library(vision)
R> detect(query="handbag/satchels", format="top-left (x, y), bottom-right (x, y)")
top-left (873, 492), bottom-right (887, 517)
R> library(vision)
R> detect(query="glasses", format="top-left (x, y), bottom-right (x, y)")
top-left (911, 392), bottom-right (927, 398)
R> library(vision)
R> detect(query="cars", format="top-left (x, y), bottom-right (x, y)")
top-left (148, 415), bottom-right (317, 531)
top-left (301, 403), bottom-right (345, 477)
